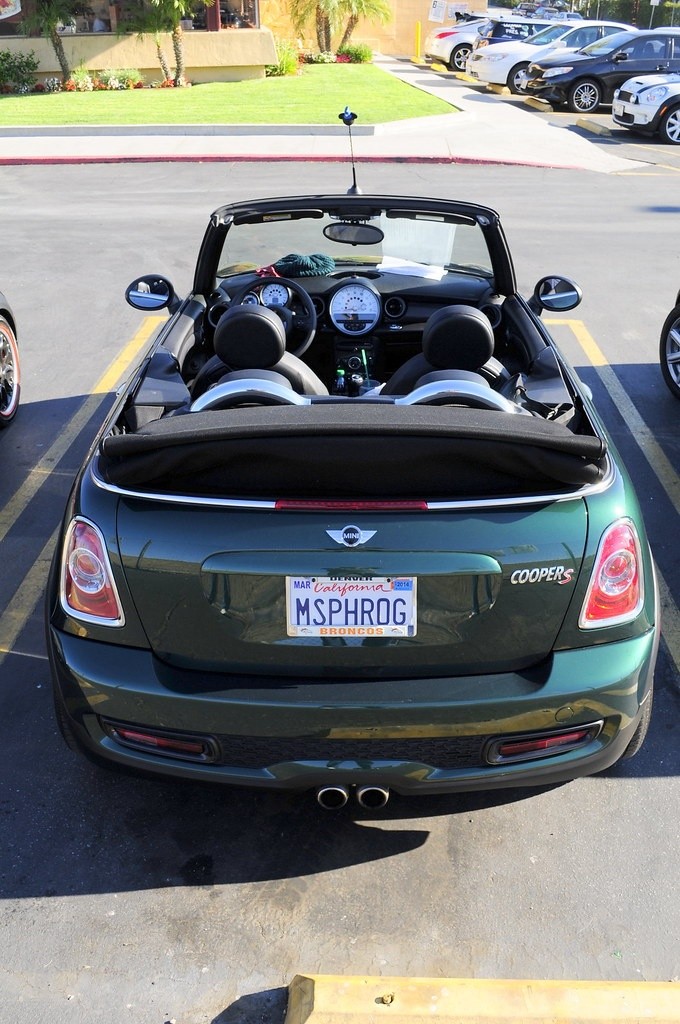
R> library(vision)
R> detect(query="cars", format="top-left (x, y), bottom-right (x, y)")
top-left (552, 12), bottom-right (583, 21)
top-left (0, 290), bottom-right (22, 426)
top-left (531, 7), bottom-right (559, 20)
top-left (46, 194), bottom-right (659, 809)
top-left (660, 287), bottom-right (680, 397)
top-left (612, 73), bottom-right (680, 145)
top-left (466, 20), bottom-right (665, 96)
top-left (520, 30), bottom-right (680, 113)
top-left (512, 3), bottom-right (540, 18)
top-left (472, 17), bottom-right (554, 50)
top-left (424, 18), bottom-right (492, 71)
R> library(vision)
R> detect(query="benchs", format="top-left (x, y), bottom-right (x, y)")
top-left (173, 369), bottom-right (529, 415)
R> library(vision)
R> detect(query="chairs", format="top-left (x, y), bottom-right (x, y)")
top-left (573, 30), bottom-right (596, 46)
top-left (380, 306), bottom-right (508, 396)
top-left (636, 42), bottom-right (666, 58)
top-left (188, 303), bottom-right (329, 397)
